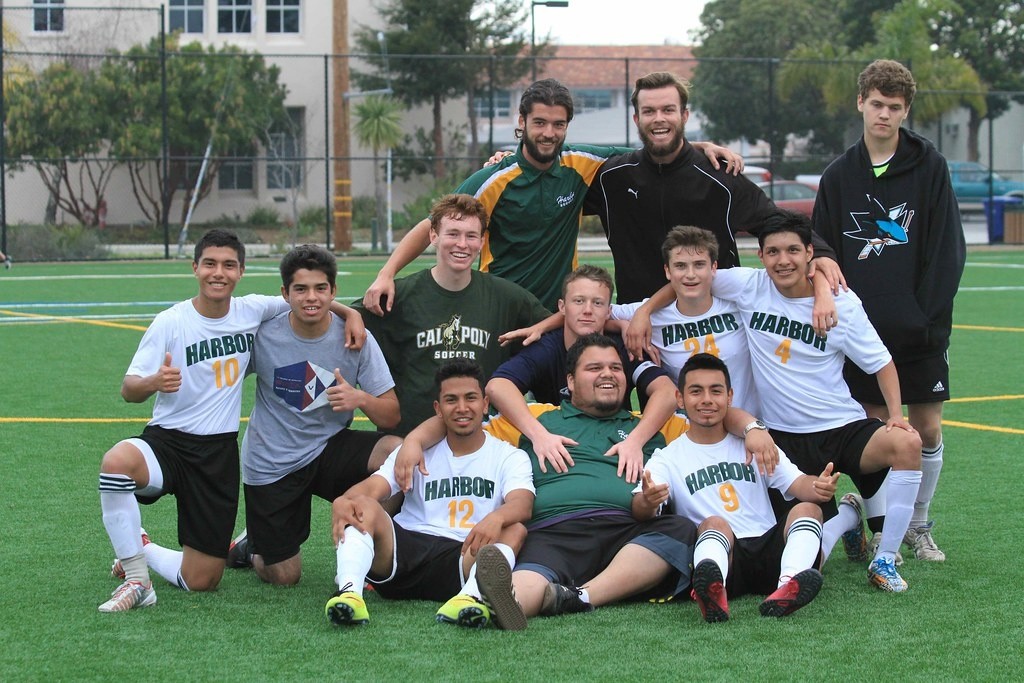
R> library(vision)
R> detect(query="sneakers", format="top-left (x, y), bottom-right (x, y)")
top-left (110, 526), bottom-right (151, 579)
top-left (870, 532), bottom-right (904, 566)
top-left (98, 579), bottom-right (158, 613)
top-left (758, 569), bottom-right (824, 618)
top-left (838, 493), bottom-right (869, 562)
top-left (903, 520), bottom-right (946, 561)
top-left (325, 582), bottom-right (370, 626)
top-left (226, 529), bottom-right (251, 569)
top-left (691, 558), bottom-right (729, 623)
top-left (867, 556), bottom-right (908, 593)
top-left (435, 594), bottom-right (490, 629)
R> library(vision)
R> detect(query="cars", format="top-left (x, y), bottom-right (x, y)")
top-left (946, 162), bottom-right (1024, 201)
top-left (735, 165), bottom-right (819, 237)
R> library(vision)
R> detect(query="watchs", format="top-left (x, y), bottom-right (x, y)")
top-left (742, 421), bottom-right (767, 436)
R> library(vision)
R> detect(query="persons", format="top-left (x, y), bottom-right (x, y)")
top-left (394, 334), bottom-right (780, 628)
top-left (325, 367), bottom-right (538, 626)
top-left (629, 352), bottom-right (841, 618)
top-left (96, 230), bottom-right (366, 609)
top-left (809, 56), bottom-right (968, 563)
top-left (228, 240), bottom-right (408, 584)
top-left (341, 190), bottom-right (554, 440)
top-left (482, 72), bottom-right (849, 304)
top-left (630, 213), bottom-right (924, 594)
top-left (499, 225), bottom-right (837, 431)
top-left (481, 263), bottom-right (677, 483)
top-left (360, 77), bottom-right (749, 315)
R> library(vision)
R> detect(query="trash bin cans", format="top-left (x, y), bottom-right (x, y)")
top-left (984, 196), bottom-right (1023, 244)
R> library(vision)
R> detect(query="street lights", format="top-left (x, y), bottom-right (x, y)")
top-left (531, 0), bottom-right (568, 83)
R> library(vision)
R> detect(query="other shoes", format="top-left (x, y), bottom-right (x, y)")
top-left (475, 544), bottom-right (528, 631)
top-left (538, 582), bottom-right (593, 617)
